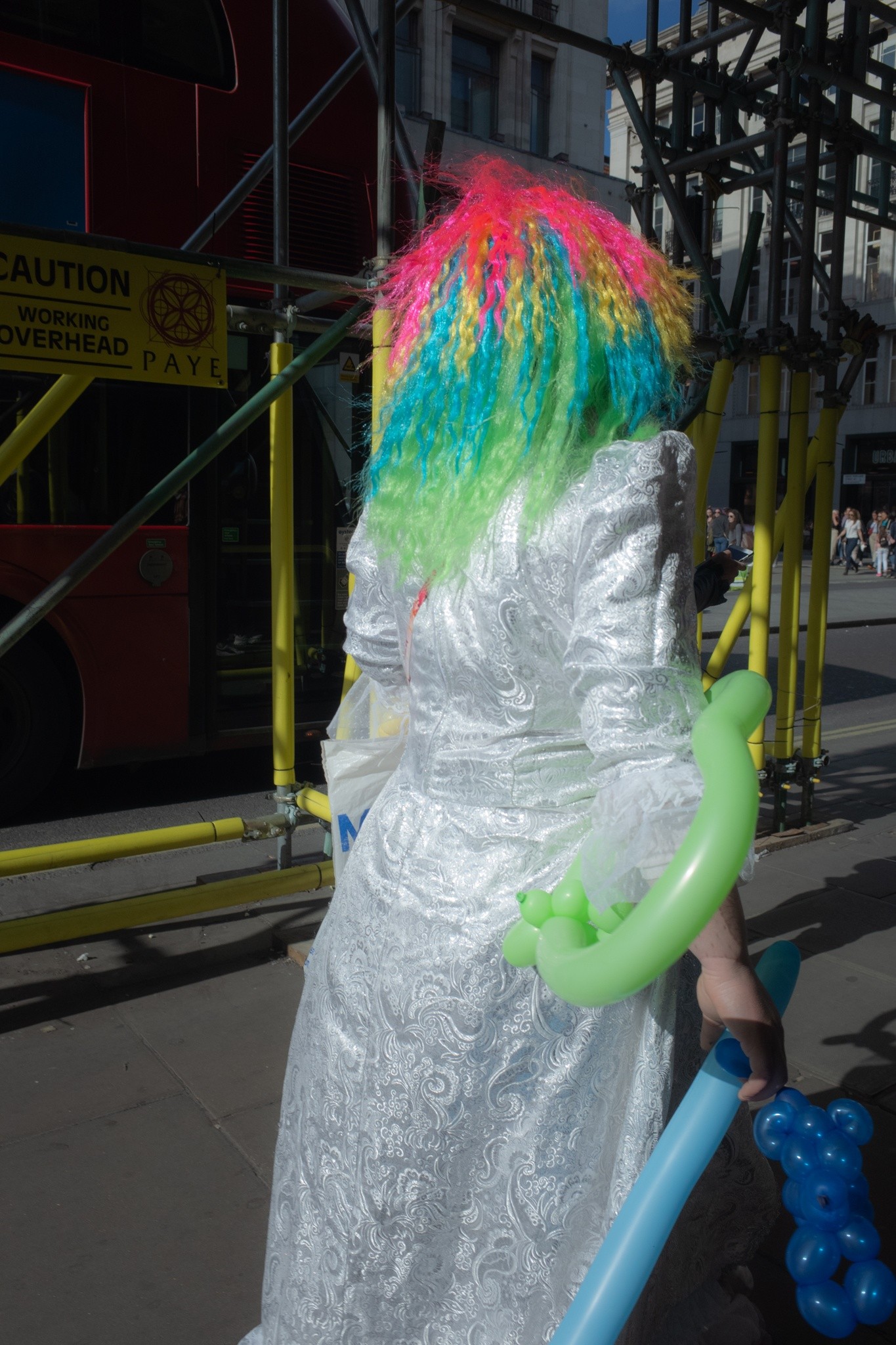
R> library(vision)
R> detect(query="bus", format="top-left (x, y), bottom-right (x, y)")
top-left (0, 0), bottom-right (414, 817)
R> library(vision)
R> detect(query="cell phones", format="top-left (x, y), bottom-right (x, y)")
top-left (726, 544), bottom-right (755, 563)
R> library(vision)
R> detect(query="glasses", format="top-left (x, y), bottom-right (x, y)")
top-left (848, 514), bottom-right (854, 516)
top-left (714, 512), bottom-right (720, 514)
top-left (727, 515), bottom-right (734, 518)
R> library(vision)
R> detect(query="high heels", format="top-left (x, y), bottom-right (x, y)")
top-left (844, 568), bottom-right (851, 575)
top-left (854, 564), bottom-right (861, 573)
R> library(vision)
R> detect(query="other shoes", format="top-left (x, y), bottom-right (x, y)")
top-left (830, 562), bottom-right (835, 565)
top-left (876, 573), bottom-right (882, 577)
top-left (837, 559), bottom-right (842, 565)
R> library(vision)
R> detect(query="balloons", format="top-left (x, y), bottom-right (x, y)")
top-left (552, 940), bottom-right (800, 1345)
top-left (713, 1037), bottom-right (896, 1339)
top-left (503, 672), bottom-right (773, 1005)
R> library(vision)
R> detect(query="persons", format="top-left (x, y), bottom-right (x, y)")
top-left (707, 507), bottom-right (896, 578)
top-left (235, 159), bottom-right (792, 1345)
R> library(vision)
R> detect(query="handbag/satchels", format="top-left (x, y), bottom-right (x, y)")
top-left (880, 539), bottom-right (889, 547)
